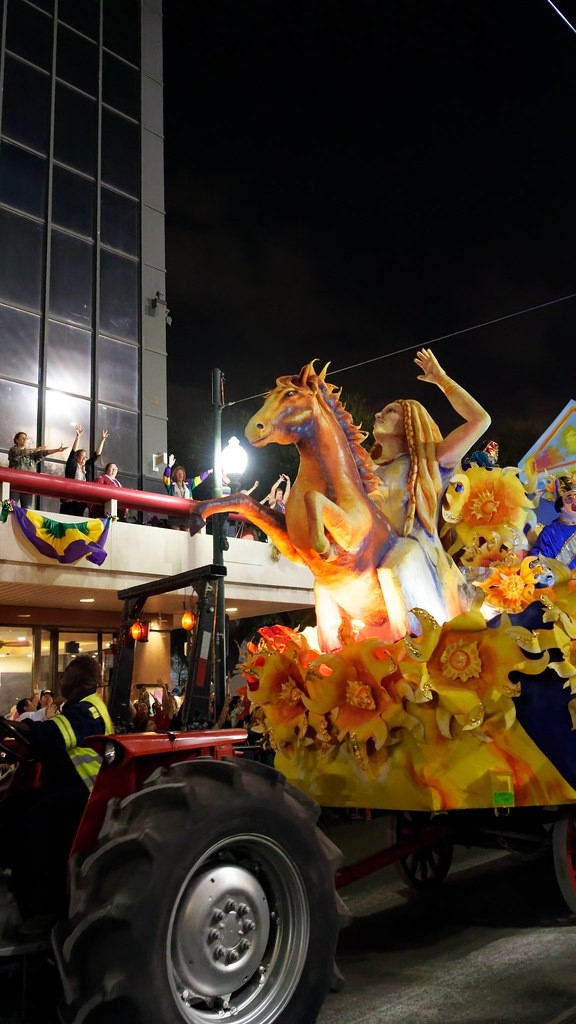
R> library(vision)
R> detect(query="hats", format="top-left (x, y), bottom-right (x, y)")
top-left (543, 469), bottom-right (576, 502)
top-left (41, 690), bottom-right (55, 697)
top-left (172, 466), bottom-right (186, 481)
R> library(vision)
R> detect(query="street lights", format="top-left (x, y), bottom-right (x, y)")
top-left (214, 437), bottom-right (248, 730)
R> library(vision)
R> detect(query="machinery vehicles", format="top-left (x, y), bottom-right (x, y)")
top-left (0, 564), bottom-right (349, 1024)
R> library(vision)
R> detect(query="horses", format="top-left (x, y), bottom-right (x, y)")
top-left (190, 357), bottom-right (474, 657)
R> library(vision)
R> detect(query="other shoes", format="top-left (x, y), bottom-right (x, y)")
top-left (16, 913), bottom-right (58, 935)
top-left (350, 813), bottom-right (364, 819)
top-left (326, 814), bottom-right (339, 819)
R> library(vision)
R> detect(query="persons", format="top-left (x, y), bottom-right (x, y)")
top-left (0, 655), bottom-right (277, 934)
top-left (529, 477), bottom-right (576, 586)
top-left (463, 442), bottom-right (498, 471)
top-left (365, 347), bottom-right (490, 541)
top-left (8, 432), bottom-right (67, 509)
top-left (84, 463), bottom-right (128, 522)
top-left (60, 426), bottom-right (110, 517)
top-left (163, 453), bottom-right (213, 531)
top-left (225, 473), bottom-right (291, 544)
